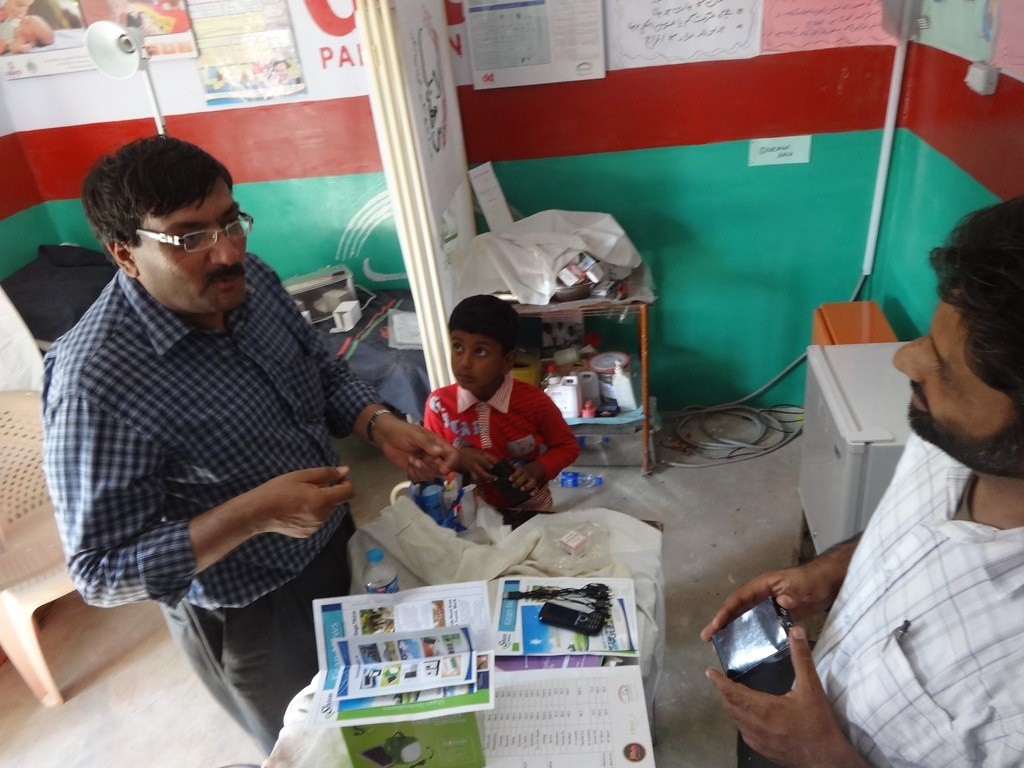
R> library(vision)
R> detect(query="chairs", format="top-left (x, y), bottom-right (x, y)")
top-left (0, 388), bottom-right (74, 706)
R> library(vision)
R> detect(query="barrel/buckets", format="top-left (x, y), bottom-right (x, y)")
top-left (542, 371), bottom-right (601, 419)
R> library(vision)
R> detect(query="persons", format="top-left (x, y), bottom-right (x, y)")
top-left (697, 192), bottom-right (1023, 768)
top-left (37, 138), bottom-right (461, 759)
top-left (424, 294), bottom-right (581, 515)
top-left (0, 0), bottom-right (55, 56)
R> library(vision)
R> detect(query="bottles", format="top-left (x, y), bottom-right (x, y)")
top-left (575, 435), bottom-right (609, 446)
top-left (549, 471), bottom-right (602, 488)
top-left (362, 548), bottom-right (400, 595)
top-left (538, 363), bottom-right (562, 389)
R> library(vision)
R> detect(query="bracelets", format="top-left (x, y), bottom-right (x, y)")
top-left (367, 409), bottom-right (393, 449)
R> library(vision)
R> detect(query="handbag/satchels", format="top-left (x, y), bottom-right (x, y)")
top-left (469, 209), bottom-right (644, 307)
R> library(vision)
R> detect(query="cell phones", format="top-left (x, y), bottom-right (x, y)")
top-left (539, 601), bottom-right (604, 636)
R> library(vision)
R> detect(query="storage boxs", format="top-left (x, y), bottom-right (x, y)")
top-left (710, 595), bottom-right (796, 684)
top-left (280, 265), bottom-right (358, 324)
top-left (329, 299), bottom-right (363, 334)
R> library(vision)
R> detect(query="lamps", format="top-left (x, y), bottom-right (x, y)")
top-left (84, 22), bottom-right (169, 136)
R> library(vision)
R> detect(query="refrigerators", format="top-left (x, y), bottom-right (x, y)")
top-left (799, 340), bottom-right (911, 555)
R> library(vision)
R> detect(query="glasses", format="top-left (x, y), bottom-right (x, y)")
top-left (135, 210), bottom-right (253, 253)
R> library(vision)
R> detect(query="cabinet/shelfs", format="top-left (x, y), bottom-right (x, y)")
top-left (509, 296), bottom-right (655, 479)
